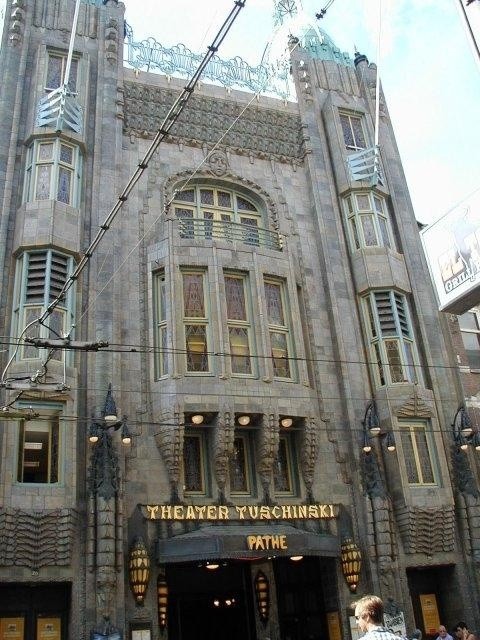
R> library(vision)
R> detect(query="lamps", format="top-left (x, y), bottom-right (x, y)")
top-left (192, 415), bottom-right (204, 424)
top-left (90, 382), bottom-right (131, 445)
top-left (362, 401), bottom-right (396, 453)
top-left (157, 572), bottom-right (168, 630)
top-left (341, 536), bottom-right (362, 592)
top-left (128, 536), bottom-right (150, 606)
top-left (237, 414), bottom-right (251, 425)
top-left (281, 417), bottom-right (293, 427)
top-left (452, 405), bottom-right (480, 451)
top-left (254, 569), bottom-right (271, 629)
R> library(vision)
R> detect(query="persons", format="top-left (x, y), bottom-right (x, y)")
top-left (354, 595), bottom-right (473, 640)
top-left (207, 149), bottom-right (228, 177)
